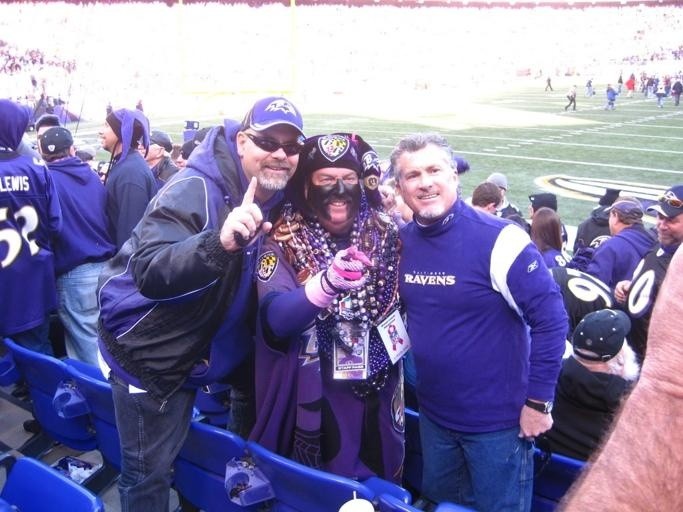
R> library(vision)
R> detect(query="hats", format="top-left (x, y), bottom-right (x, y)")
top-left (572, 309), bottom-right (632, 362)
top-left (487, 173), bottom-right (508, 192)
top-left (303, 134), bottom-right (362, 175)
top-left (106, 108), bottom-right (144, 149)
top-left (646, 185), bottom-right (683, 218)
top-left (142, 131), bottom-right (172, 153)
top-left (40, 128), bottom-right (73, 155)
top-left (37, 113), bottom-right (59, 132)
top-left (240, 96), bottom-right (307, 141)
top-left (603, 197), bottom-right (644, 220)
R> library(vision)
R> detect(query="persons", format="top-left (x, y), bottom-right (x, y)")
top-left (0, 29), bottom-right (683, 512)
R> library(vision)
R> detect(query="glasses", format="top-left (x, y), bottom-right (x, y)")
top-left (247, 133), bottom-right (303, 156)
top-left (656, 193), bottom-right (682, 209)
top-left (519, 436), bottom-right (551, 482)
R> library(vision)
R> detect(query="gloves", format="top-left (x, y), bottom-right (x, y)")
top-left (321, 246), bottom-right (372, 295)
